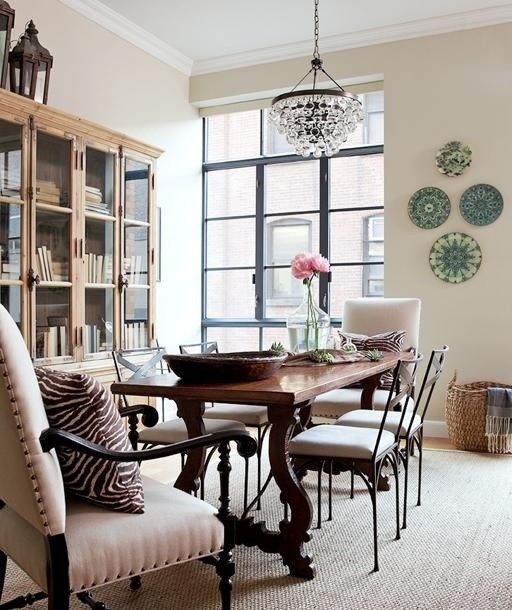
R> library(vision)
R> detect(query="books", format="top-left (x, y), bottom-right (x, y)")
top-left (0, 166), bottom-right (149, 358)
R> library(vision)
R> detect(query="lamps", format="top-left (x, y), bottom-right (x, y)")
top-left (267, 0), bottom-right (364, 158)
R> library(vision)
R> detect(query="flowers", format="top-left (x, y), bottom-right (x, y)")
top-left (289, 252), bottom-right (331, 352)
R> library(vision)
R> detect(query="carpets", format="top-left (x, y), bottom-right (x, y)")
top-left (0, 446), bottom-right (512, 610)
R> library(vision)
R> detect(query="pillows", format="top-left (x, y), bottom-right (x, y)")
top-left (33, 365), bottom-right (145, 514)
top-left (336, 329), bottom-right (409, 393)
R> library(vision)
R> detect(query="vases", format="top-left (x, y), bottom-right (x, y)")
top-left (287, 284), bottom-right (331, 355)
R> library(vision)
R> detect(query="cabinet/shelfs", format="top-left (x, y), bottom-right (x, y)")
top-left (0, 86), bottom-right (165, 435)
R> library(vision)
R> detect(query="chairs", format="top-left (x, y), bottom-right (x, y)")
top-left (282, 352), bottom-right (424, 572)
top-left (0, 304), bottom-right (258, 610)
top-left (328, 344), bottom-right (449, 529)
top-left (298, 298), bottom-right (420, 456)
top-left (179, 341), bottom-right (298, 510)
top-left (110, 347), bottom-right (249, 519)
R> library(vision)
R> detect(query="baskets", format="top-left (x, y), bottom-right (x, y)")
top-left (445, 370), bottom-right (512, 452)
top-left (162, 350), bottom-right (287, 379)
